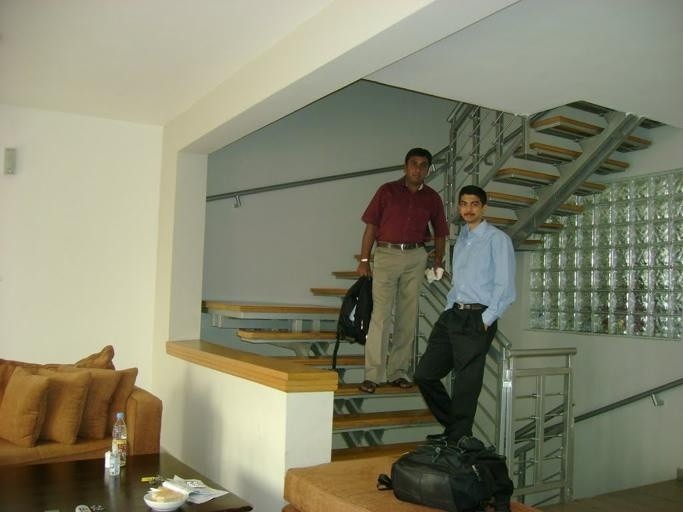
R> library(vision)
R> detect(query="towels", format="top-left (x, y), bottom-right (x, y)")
top-left (424, 267), bottom-right (446, 284)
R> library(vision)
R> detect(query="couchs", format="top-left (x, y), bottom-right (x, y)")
top-left (2, 336), bottom-right (165, 467)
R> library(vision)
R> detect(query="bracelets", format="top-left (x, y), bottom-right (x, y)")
top-left (361, 259), bottom-right (369, 262)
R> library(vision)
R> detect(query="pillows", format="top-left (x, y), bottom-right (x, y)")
top-left (1, 343), bottom-right (141, 450)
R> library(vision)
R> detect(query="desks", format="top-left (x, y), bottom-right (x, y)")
top-left (1, 452), bottom-right (255, 512)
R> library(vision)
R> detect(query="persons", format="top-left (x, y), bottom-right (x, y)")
top-left (356, 149), bottom-right (449, 393)
top-left (412, 185), bottom-right (517, 445)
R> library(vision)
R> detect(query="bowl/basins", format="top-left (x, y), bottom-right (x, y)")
top-left (143, 489), bottom-right (188, 511)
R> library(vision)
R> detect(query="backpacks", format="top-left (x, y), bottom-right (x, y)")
top-left (337, 276), bottom-right (374, 345)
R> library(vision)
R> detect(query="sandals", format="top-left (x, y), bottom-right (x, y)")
top-left (358, 378), bottom-right (413, 394)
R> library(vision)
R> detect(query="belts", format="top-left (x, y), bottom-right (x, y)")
top-left (376, 241), bottom-right (425, 250)
top-left (453, 302), bottom-right (483, 311)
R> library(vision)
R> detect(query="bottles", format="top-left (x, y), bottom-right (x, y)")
top-left (110, 411), bottom-right (127, 468)
top-left (103, 450), bottom-right (111, 468)
top-left (109, 440), bottom-right (120, 476)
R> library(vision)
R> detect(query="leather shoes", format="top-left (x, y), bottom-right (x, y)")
top-left (426, 432), bottom-right (449, 444)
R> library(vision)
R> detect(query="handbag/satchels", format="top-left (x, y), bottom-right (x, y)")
top-left (391, 434), bottom-right (514, 512)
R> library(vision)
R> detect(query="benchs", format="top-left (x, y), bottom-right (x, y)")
top-left (280, 454), bottom-right (542, 511)
